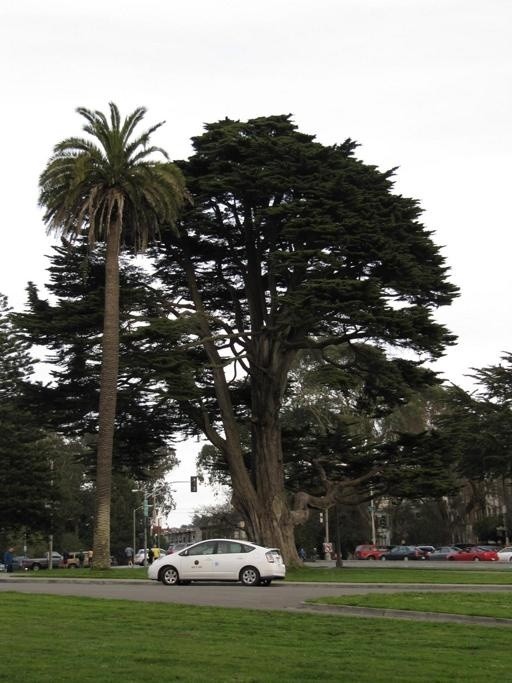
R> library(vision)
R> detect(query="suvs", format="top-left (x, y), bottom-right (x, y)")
top-left (165, 543), bottom-right (195, 555)
top-left (0, 556), bottom-right (32, 571)
top-left (133, 547), bottom-right (166, 566)
top-left (60, 551), bottom-right (95, 569)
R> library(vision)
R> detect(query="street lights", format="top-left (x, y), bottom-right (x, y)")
top-left (131, 489), bottom-right (178, 549)
top-left (132, 504), bottom-right (153, 566)
top-left (325, 460), bottom-right (349, 561)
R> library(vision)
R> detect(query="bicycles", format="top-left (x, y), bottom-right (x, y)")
top-left (127, 556), bottom-right (136, 569)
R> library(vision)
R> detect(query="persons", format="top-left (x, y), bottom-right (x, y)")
top-left (125, 544), bottom-right (134, 566)
top-left (79, 549), bottom-right (85, 567)
top-left (148, 548), bottom-right (154, 564)
top-left (63, 549), bottom-right (69, 567)
top-left (153, 543), bottom-right (161, 560)
top-left (4, 547), bottom-right (16, 572)
top-left (298, 548), bottom-right (306, 559)
top-left (89, 548), bottom-right (94, 568)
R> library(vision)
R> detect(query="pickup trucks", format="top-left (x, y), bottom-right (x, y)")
top-left (22, 550), bottom-right (65, 571)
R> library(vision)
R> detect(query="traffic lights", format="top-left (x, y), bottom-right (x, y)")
top-left (191, 476), bottom-right (197, 492)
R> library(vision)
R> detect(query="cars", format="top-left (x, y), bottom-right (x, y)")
top-left (149, 537), bottom-right (287, 586)
top-left (354, 544), bottom-right (511, 563)
